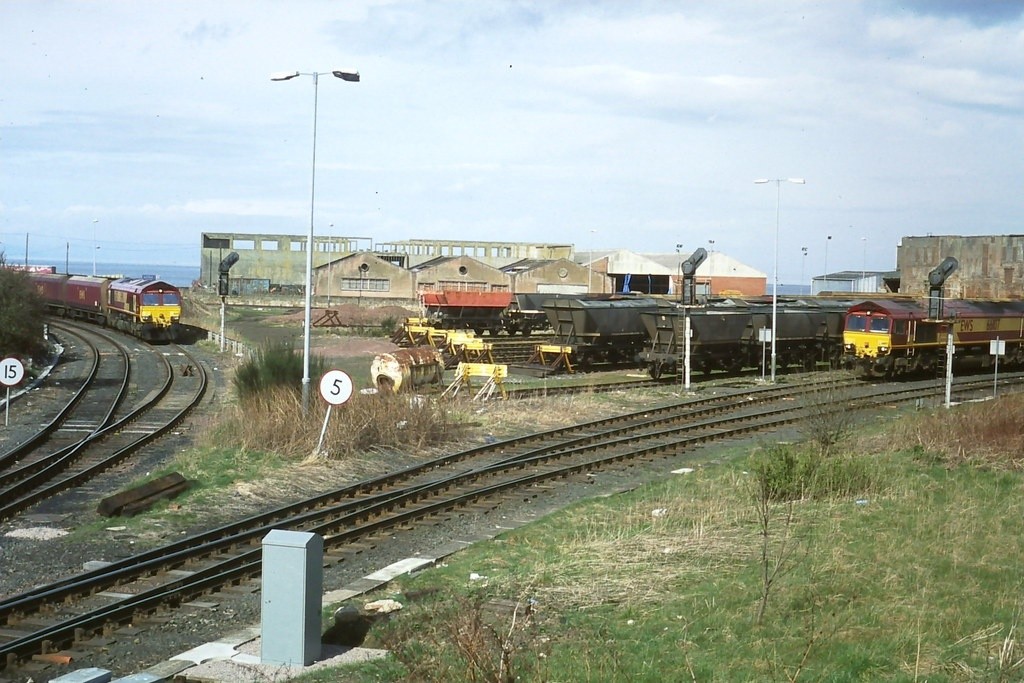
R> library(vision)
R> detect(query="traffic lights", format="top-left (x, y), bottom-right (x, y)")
top-left (218, 273), bottom-right (228, 298)
top-left (929, 288), bottom-right (943, 320)
top-left (681, 277), bottom-right (695, 307)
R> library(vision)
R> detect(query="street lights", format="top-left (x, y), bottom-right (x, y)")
top-left (823, 234), bottom-right (832, 294)
top-left (675, 243), bottom-right (683, 305)
top-left (589, 229), bottom-right (597, 294)
top-left (269, 68), bottom-right (365, 421)
top-left (861, 235), bottom-right (868, 290)
top-left (799, 246), bottom-right (809, 293)
top-left (90, 219), bottom-right (101, 277)
top-left (707, 240), bottom-right (716, 303)
top-left (752, 177), bottom-right (807, 383)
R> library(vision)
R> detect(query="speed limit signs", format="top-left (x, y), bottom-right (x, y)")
top-left (0, 358), bottom-right (24, 387)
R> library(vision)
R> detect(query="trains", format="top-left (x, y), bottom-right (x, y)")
top-left (4, 269), bottom-right (184, 345)
top-left (840, 296), bottom-right (1024, 382)
